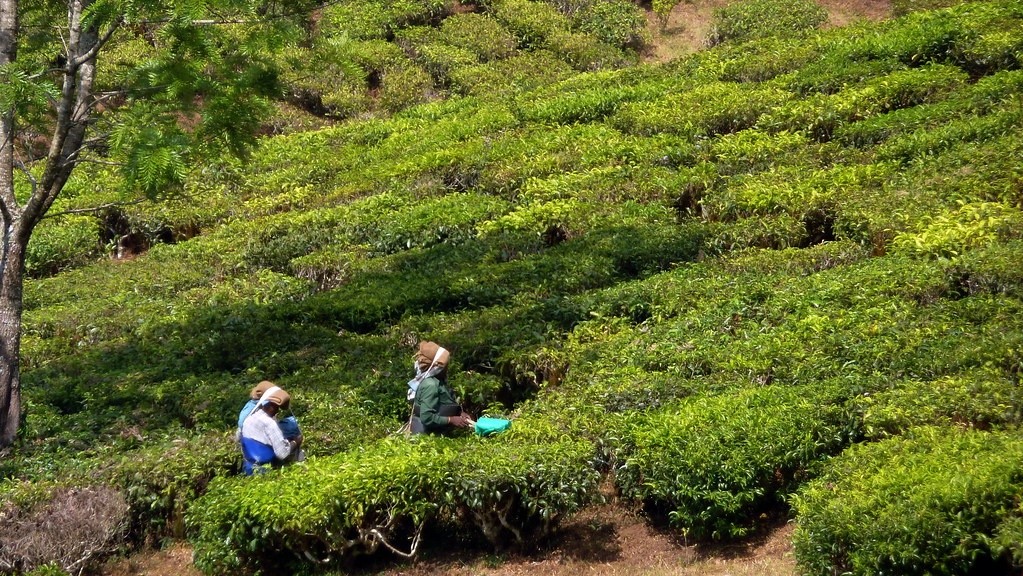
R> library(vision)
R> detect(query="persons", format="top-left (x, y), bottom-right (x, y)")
top-left (238, 381), bottom-right (303, 476)
top-left (407, 341), bottom-right (471, 439)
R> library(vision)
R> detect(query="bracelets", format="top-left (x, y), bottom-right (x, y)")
top-left (292, 440), bottom-right (297, 446)
top-left (448, 416), bottom-right (450, 424)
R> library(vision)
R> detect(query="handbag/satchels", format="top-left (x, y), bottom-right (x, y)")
top-left (277, 416), bottom-right (300, 438)
top-left (465, 417), bottom-right (511, 435)
